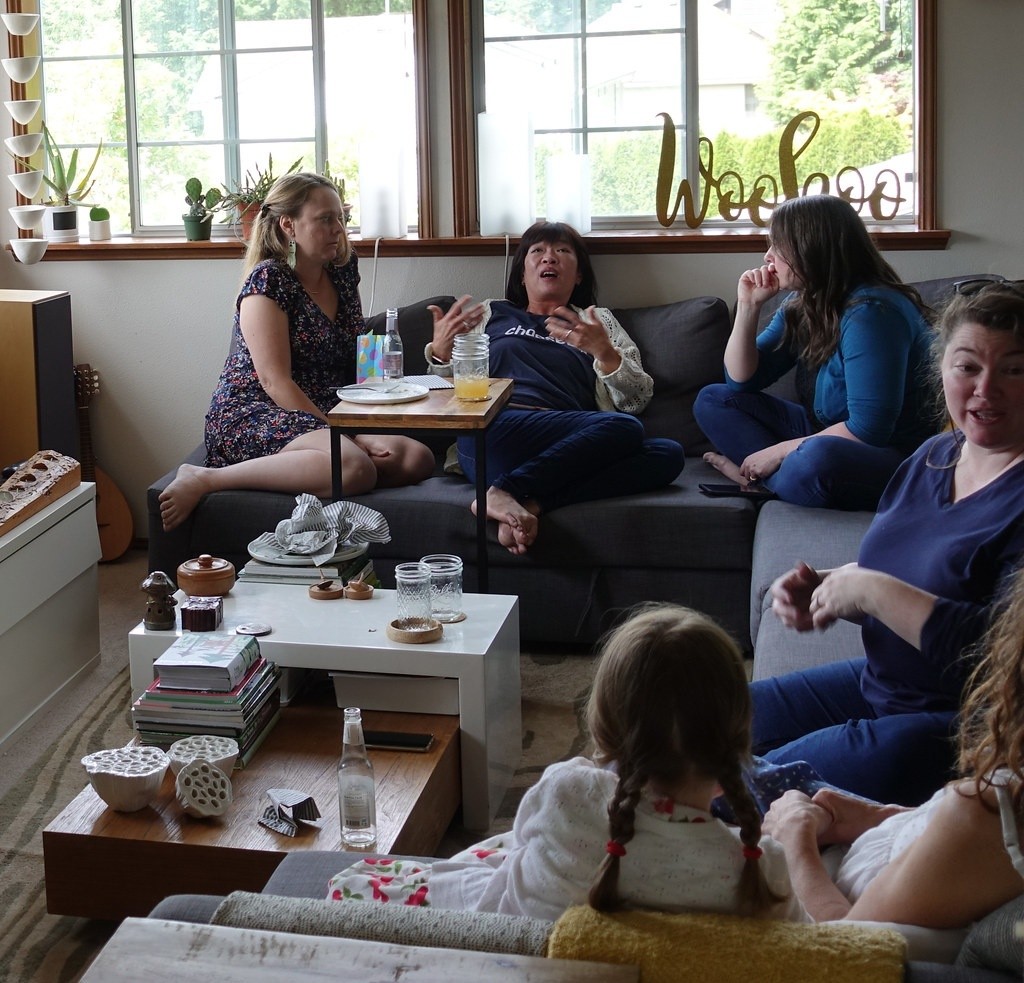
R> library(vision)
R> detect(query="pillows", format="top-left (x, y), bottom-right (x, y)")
top-left (789, 274), bottom-right (1006, 436)
top-left (362, 292), bottom-right (462, 375)
top-left (610, 295), bottom-right (732, 457)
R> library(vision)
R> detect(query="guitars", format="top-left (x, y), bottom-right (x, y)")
top-left (74, 364), bottom-right (136, 564)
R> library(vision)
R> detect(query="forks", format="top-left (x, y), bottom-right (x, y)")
top-left (329, 384), bottom-right (400, 393)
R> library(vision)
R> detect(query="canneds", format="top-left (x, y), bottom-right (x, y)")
top-left (451, 333), bottom-right (490, 401)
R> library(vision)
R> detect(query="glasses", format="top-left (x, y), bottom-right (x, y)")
top-left (953, 278), bottom-right (1021, 294)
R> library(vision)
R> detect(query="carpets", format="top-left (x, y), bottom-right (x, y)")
top-left (0, 641), bottom-right (751, 983)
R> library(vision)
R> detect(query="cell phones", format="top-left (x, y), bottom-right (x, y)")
top-left (699, 483), bottom-right (771, 497)
top-left (363, 732), bottom-right (435, 752)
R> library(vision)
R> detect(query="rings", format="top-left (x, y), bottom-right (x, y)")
top-left (566, 329), bottom-right (573, 337)
top-left (750, 476), bottom-right (757, 480)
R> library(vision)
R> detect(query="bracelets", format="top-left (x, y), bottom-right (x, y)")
top-left (432, 356), bottom-right (451, 365)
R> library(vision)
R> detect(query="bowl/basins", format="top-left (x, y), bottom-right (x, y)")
top-left (3, 13), bottom-right (49, 264)
top-left (177, 554), bottom-right (236, 597)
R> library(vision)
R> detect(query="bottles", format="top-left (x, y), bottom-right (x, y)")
top-left (382, 308), bottom-right (404, 383)
top-left (338, 707), bottom-right (377, 848)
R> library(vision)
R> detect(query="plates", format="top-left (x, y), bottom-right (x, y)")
top-left (336, 383), bottom-right (429, 404)
top-left (247, 536), bottom-right (371, 565)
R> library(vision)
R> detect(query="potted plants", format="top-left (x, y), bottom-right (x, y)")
top-left (89, 205), bottom-right (111, 240)
top-left (5, 119), bottom-right (105, 242)
top-left (318, 159), bottom-right (353, 236)
top-left (182, 177), bottom-right (222, 241)
top-left (222, 155), bottom-right (304, 241)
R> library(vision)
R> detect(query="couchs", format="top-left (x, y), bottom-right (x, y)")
top-left (76, 266), bottom-right (1024, 983)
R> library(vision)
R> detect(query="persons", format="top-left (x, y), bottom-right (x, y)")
top-left (694, 197), bottom-right (960, 506)
top-left (159, 172), bottom-right (434, 531)
top-left (326, 601), bottom-right (816, 928)
top-left (761, 573), bottom-right (1024, 931)
top-left (425, 221), bottom-right (684, 556)
top-left (717, 274), bottom-right (1024, 803)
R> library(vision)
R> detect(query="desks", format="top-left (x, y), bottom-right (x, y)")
top-left (42, 581), bottom-right (522, 917)
top-left (325, 377), bottom-right (515, 585)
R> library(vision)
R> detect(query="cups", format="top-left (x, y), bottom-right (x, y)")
top-left (394, 562), bottom-right (431, 629)
top-left (451, 333), bottom-right (490, 401)
top-left (419, 553), bottom-right (466, 623)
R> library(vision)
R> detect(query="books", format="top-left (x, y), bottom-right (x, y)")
top-left (131, 631), bottom-right (285, 771)
